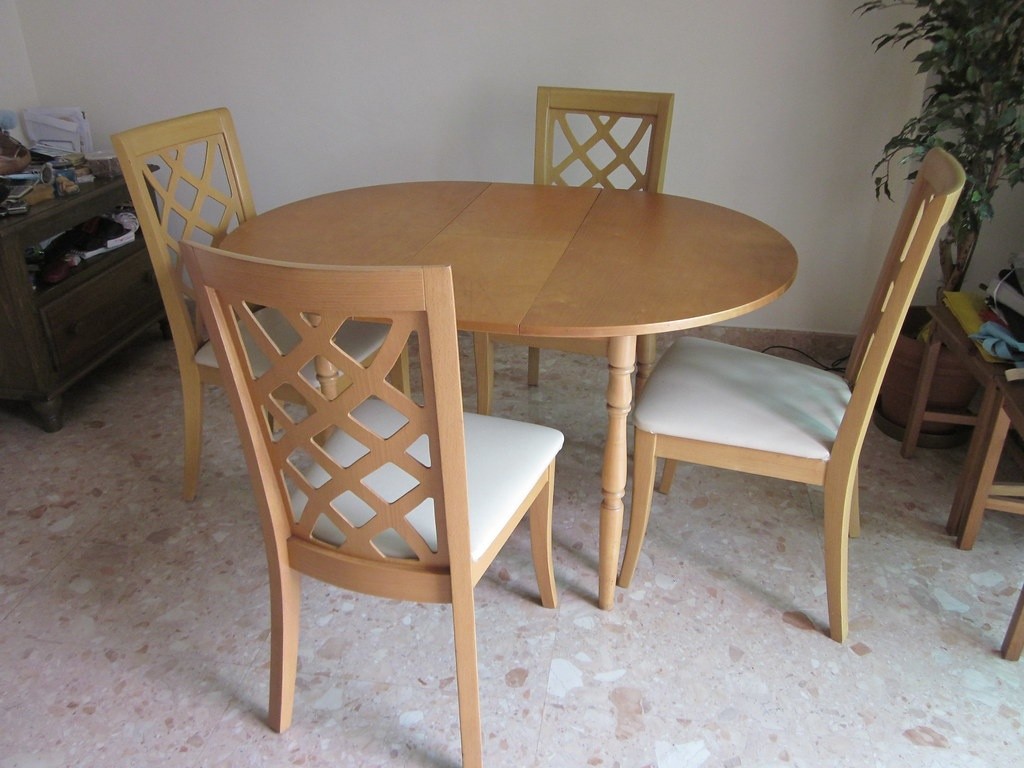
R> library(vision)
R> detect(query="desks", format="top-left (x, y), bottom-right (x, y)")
top-left (218, 181), bottom-right (799, 612)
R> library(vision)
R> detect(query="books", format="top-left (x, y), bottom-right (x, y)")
top-left (102, 228), bottom-right (135, 248)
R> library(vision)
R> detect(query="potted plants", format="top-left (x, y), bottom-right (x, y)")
top-left (851, 0), bottom-right (1024, 451)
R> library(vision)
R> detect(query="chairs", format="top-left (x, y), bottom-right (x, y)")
top-left (109, 107), bottom-right (413, 504)
top-left (617, 147), bottom-right (966, 643)
top-left (177, 238), bottom-right (564, 768)
top-left (473, 85), bottom-right (675, 416)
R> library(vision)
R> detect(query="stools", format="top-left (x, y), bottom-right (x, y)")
top-left (955, 375), bottom-right (1024, 661)
top-left (898, 304), bottom-right (1024, 538)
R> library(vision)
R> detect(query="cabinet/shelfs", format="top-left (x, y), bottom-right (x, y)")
top-left (0, 158), bottom-right (173, 434)
